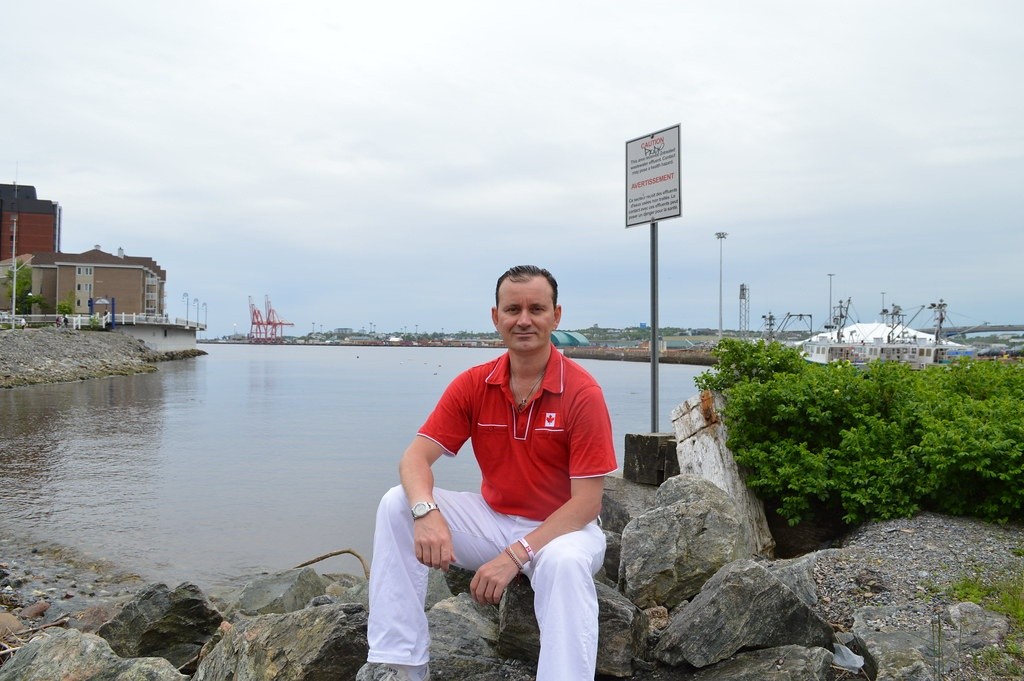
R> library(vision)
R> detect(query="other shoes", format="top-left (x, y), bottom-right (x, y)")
top-left (375, 664), bottom-right (430, 680)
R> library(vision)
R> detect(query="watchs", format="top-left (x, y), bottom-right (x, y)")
top-left (410, 501), bottom-right (441, 522)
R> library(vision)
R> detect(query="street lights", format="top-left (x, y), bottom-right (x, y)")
top-left (714, 231), bottom-right (730, 341)
top-left (22, 289), bottom-right (32, 318)
top-left (827, 273), bottom-right (836, 332)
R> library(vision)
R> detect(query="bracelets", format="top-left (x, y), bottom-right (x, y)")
top-left (504, 544), bottom-right (523, 570)
top-left (517, 538), bottom-right (534, 562)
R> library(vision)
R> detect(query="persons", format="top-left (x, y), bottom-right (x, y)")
top-left (20, 316), bottom-right (26, 330)
top-left (355, 266), bottom-right (621, 681)
top-left (56, 315), bottom-right (69, 328)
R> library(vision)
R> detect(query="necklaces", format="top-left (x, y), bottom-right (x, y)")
top-left (510, 370), bottom-right (546, 410)
